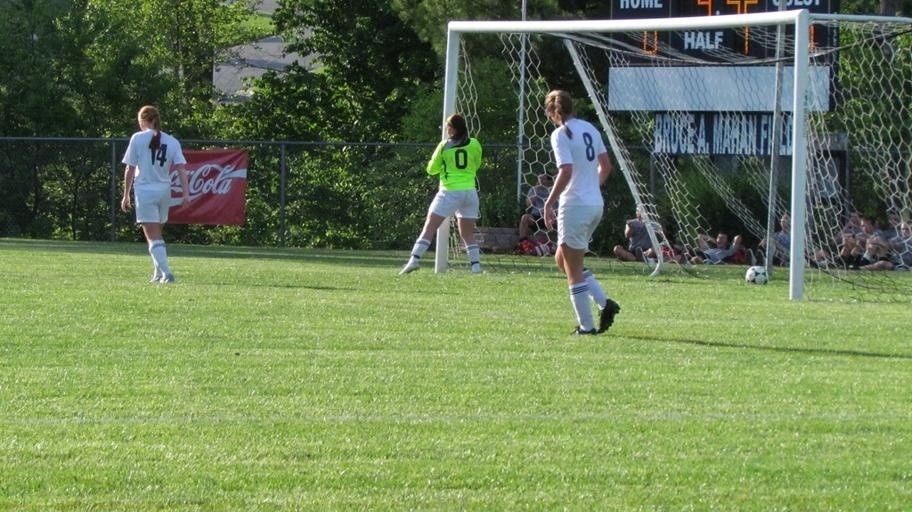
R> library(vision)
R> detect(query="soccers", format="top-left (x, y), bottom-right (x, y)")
top-left (746, 266), bottom-right (767, 284)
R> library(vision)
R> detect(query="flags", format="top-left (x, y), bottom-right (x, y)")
top-left (165, 147), bottom-right (250, 228)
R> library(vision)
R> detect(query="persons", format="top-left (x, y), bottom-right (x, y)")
top-left (613, 202), bottom-right (665, 268)
top-left (512, 173), bottom-right (561, 256)
top-left (756, 211), bottom-right (790, 268)
top-left (119, 104), bottom-right (192, 285)
top-left (659, 230), bottom-right (743, 265)
top-left (854, 220), bottom-right (912, 271)
top-left (825, 212), bottom-right (886, 271)
top-left (543, 87), bottom-right (622, 335)
top-left (392, 113), bottom-right (487, 277)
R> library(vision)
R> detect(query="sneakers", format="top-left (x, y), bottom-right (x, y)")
top-left (635, 245), bottom-right (651, 264)
top-left (399, 261), bottom-right (421, 275)
top-left (150, 274), bottom-right (175, 283)
top-left (745, 248), bottom-right (766, 266)
top-left (598, 298), bottom-right (621, 333)
top-left (570, 327), bottom-right (597, 335)
